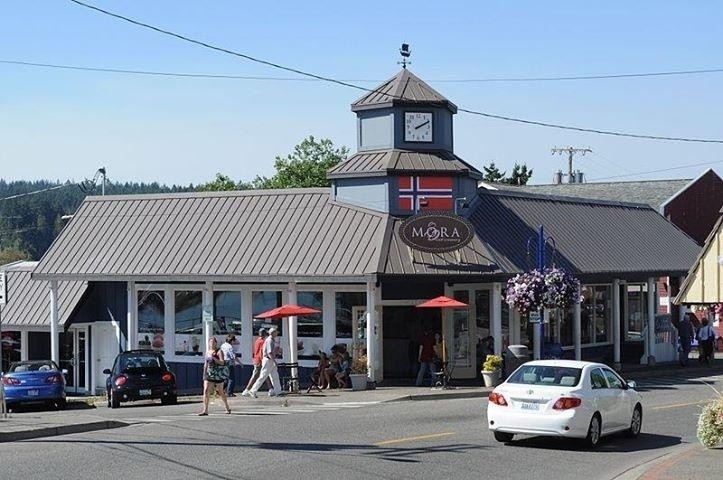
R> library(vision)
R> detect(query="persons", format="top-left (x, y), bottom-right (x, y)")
top-left (198, 337), bottom-right (231, 416)
top-left (415, 325), bottom-right (437, 386)
top-left (433, 331), bottom-right (448, 384)
top-left (248, 327), bottom-right (287, 398)
top-left (678, 315), bottom-right (715, 368)
top-left (310, 343), bottom-right (352, 389)
top-left (241, 328), bottom-right (275, 396)
top-left (220, 335), bottom-right (243, 397)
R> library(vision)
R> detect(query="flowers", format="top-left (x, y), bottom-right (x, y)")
top-left (347, 354), bottom-right (369, 375)
top-left (501, 266), bottom-right (585, 315)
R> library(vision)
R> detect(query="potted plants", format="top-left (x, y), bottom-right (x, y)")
top-left (479, 353), bottom-right (504, 389)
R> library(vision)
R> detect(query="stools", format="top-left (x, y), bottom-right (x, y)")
top-left (302, 368), bottom-right (350, 394)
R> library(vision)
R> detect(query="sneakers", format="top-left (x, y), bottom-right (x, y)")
top-left (226, 387), bottom-right (285, 398)
top-left (198, 410), bottom-right (209, 417)
top-left (225, 408), bottom-right (231, 415)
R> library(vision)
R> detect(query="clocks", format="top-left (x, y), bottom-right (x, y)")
top-left (402, 111), bottom-right (435, 144)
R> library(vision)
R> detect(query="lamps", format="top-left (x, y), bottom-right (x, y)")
top-left (415, 196), bottom-right (429, 213)
top-left (453, 196), bottom-right (469, 216)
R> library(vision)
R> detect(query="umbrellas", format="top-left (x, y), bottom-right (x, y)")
top-left (416, 295), bottom-right (472, 386)
top-left (253, 304), bottom-right (322, 392)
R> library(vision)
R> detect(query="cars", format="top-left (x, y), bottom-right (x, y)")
top-left (1, 358), bottom-right (71, 408)
top-left (99, 347), bottom-right (180, 409)
top-left (484, 357), bottom-right (644, 448)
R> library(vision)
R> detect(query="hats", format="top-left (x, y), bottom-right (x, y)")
top-left (227, 334), bottom-right (238, 341)
top-left (259, 328), bottom-right (268, 337)
top-left (268, 327), bottom-right (279, 334)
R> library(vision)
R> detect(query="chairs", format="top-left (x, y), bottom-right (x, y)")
top-left (431, 360), bottom-right (458, 390)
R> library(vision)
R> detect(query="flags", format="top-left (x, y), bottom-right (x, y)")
top-left (397, 175), bottom-right (454, 212)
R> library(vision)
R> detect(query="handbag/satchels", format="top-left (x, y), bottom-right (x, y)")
top-left (205, 348), bottom-right (230, 384)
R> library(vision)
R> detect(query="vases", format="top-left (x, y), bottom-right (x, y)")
top-left (348, 373), bottom-right (368, 390)
